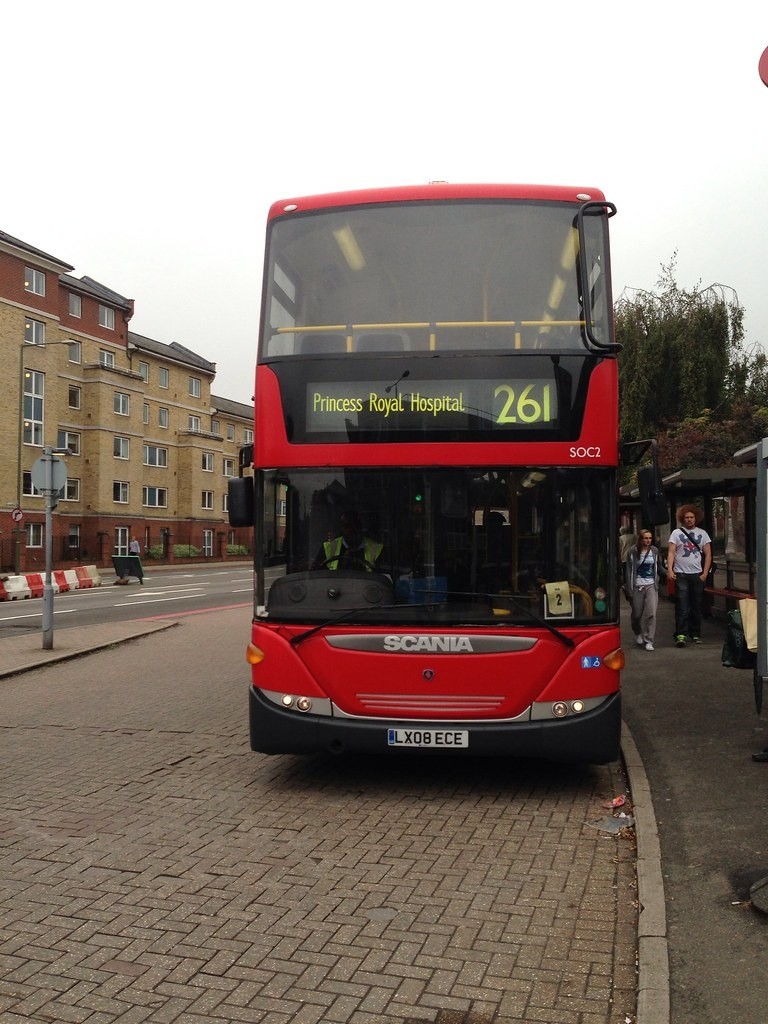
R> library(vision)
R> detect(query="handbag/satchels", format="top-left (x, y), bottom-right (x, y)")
top-left (621, 582), bottom-right (629, 600)
top-left (700, 553), bottom-right (717, 577)
top-left (722, 599), bottom-right (758, 669)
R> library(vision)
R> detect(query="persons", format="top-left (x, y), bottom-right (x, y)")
top-left (129, 535), bottom-right (140, 555)
top-left (667, 505), bottom-right (713, 648)
top-left (619, 526), bottom-right (639, 583)
top-left (483, 512), bottom-right (507, 530)
top-left (626, 529), bottom-right (677, 650)
top-left (309, 511), bottom-right (396, 583)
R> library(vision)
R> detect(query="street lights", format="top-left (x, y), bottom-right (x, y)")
top-left (14, 340), bottom-right (78, 575)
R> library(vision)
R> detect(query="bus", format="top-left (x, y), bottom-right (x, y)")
top-left (228, 179), bottom-right (671, 771)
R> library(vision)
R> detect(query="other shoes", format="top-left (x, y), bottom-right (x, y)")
top-left (752, 748), bottom-right (768, 762)
top-left (645, 643), bottom-right (654, 651)
top-left (635, 634), bottom-right (643, 644)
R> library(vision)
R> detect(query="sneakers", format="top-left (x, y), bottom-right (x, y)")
top-left (676, 634), bottom-right (686, 646)
top-left (691, 633), bottom-right (702, 644)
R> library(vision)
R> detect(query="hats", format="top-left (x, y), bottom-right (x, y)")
top-left (677, 504), bottom-right (704, 525)
